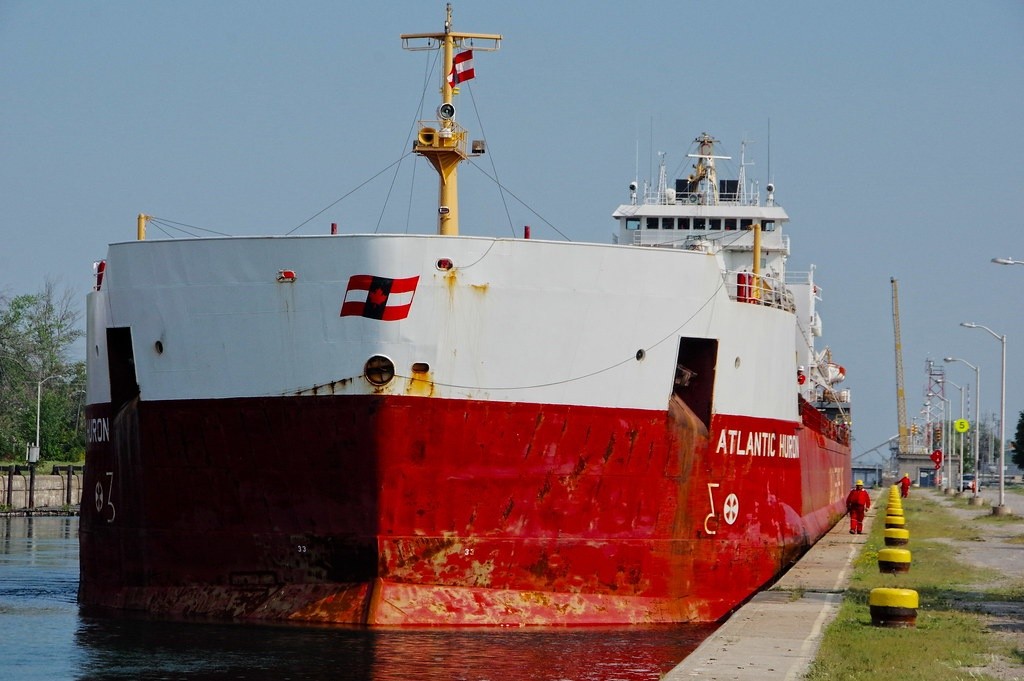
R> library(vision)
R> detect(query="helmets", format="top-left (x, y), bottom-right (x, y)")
top-left (904, 473), bottom-right (908, 477)
top-left (856, 480), bottom-right (864, 485)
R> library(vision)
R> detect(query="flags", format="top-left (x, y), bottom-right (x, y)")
top-left (447, 49), bottom-right (475, 88)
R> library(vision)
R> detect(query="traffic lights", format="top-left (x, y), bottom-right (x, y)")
top-left (936, 428), bottom-right (941, 442)
top-left (912, 425), bottom-right (918, 436)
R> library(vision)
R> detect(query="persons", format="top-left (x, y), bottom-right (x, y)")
top-left (895, 473), bottom-right (910, 498)
top-left (797, 365), bottom-right (806, 393)
top-left (846, 479), bottom-right (871, 534)
top-left (841, 421), bottom-right (847, 429)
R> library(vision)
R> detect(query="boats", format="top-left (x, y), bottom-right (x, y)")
top-left (74, 1), bottom-right (852, 631)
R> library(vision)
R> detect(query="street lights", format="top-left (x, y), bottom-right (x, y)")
top-left (927, 392), bottom-right (950, 490)
top-left (923, 403), bottom-right (945, 490)
top-left (943, 357), bottom-right (980, 496)
top-left (936, 379), bottom-right (964, 493)
top-left (912, 416), bottom-right (933, 454)
top-left (960, 323), bottom-right (1006, 506)
top-left (36, 374), bottom-right (62, 447)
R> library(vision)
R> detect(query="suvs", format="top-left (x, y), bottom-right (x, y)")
top-left (957, 474), bottom-right (981, 492)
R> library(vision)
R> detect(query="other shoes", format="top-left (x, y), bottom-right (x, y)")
top-left (857, 531), bottom-right (863, 534)
top-left (904, 496), bottom-right (907, 498)
top-left (901, 494), bottom-right (903, 497)
top-left (849, 529), bottom-right (855, 534)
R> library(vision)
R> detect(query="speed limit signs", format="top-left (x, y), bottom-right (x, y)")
top-left (955, 419), bottom-right (970, 433)
